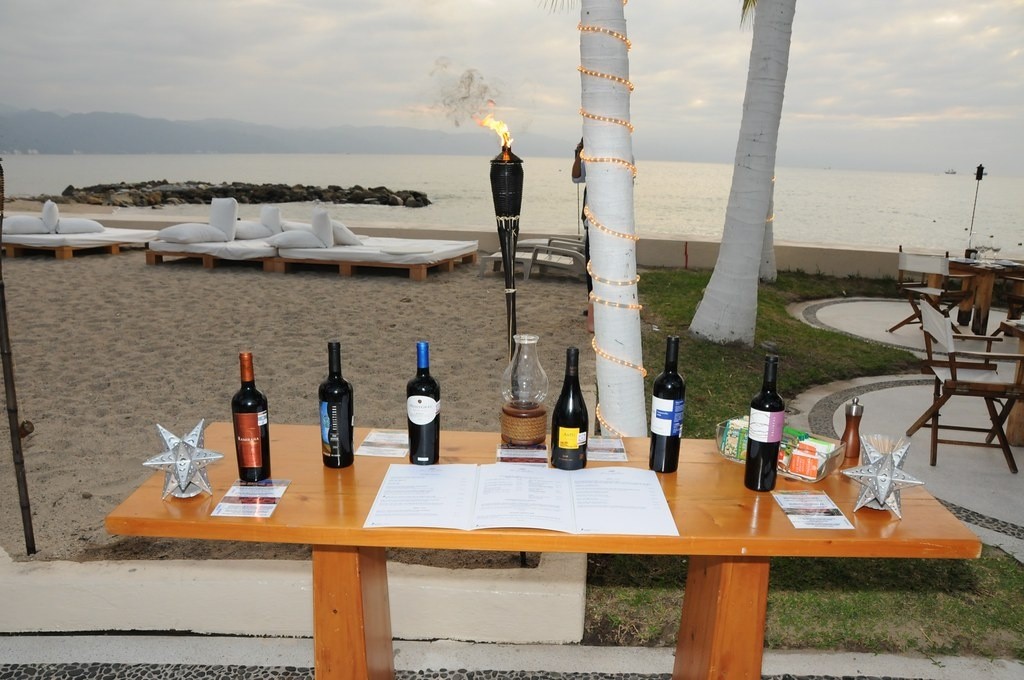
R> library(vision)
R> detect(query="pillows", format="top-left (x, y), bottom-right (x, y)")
top-left (42, 199), bottom-right (58, 234)
top-left (313, 206), bottom-right (335, 249)
top-left (155, 222), bottom-right (227, 244)
top-left (281, 219), bottom-right (314, 232)
top-left (209, 198), bottom-right (238, 241)
top-left (56, 218), bottom-right (105, 233)
top-left (330, 219), bottom-right (364, 245)
top-left (2, 214), bottom-right (50, 234)
top-left (265, 230), bottom-right (327, 248)
top-left (235, 221), bottom-right (274, 239)
top-left (260, 207), bottom-right (283, 235)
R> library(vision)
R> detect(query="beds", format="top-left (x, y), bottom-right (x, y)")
top-left (146, 235), bottom-right (368, 271)
top-left (273, 237), bottom-right (479, 280)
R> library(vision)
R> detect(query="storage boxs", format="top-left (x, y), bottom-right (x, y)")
top-left (716, 415), bottom-right (847, 484)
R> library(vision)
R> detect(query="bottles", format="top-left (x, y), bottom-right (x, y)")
top-left (318, 342), bottom-right (354, 469)
top-left (406, 341), bottom-right (442, 466)
top-left (648, 333), bottom-right (687, 473)
top-left (743, 354), bottom-right (786, 494)
top-left (550, 346), bottom-right (589, 472)
top-left (231, 350), bottom-right (272, 483)
top-left (839, 397), bottom-right (864, 460)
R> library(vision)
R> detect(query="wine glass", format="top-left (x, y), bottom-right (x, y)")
top-left (971, 232), bottom-right (1003, 264)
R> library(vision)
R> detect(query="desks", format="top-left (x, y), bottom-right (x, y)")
top-left (947, 259), bottom-right (1024, 336)
top-left (999, 320), bottom-right (1024, 446)
top-left (102, 420), bottom-right (983, 680)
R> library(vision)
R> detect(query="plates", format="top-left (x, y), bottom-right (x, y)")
top-left (1001, 261), bottom-right (1022, 267)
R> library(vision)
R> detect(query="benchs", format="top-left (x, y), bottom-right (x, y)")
top-left (2, 227), bottom-right (158, 260)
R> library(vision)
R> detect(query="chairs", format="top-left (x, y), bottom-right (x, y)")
top-left (989, 274), bottom-right (1024, 337)
top-left (499, 229), bottom-right (587, 253)
top-left (889, 245), bottom-right (977, 343)
top-left (905, 293), bottom-right (1024, 473)
top-left (480, 241), bottom-right (589, 283)
